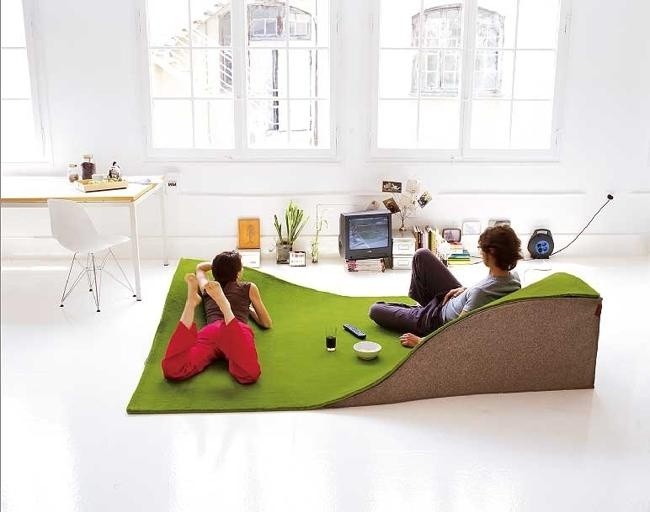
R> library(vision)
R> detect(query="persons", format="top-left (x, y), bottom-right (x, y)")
top-left (368, 225), bottom-right (523, 349)
top-left (161, 250), bottom-right (272, 384)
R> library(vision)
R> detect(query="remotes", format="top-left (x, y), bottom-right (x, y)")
top-left (343, 323), bottom-right (365, 339)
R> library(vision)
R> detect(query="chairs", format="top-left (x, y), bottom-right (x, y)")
top-left (47, 198), bottom-right (137, 313)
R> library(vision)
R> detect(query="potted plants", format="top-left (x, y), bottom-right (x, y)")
top-left (273, 199), bottom-right (329, 264)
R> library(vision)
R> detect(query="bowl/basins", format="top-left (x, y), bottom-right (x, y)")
top-left (353, 341), bottom-right (382, 360)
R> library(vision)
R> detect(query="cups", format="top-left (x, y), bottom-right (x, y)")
top-left (92, 174), bottom-right (104, 182)
top-left (325, 326), bottom-right (337, 352)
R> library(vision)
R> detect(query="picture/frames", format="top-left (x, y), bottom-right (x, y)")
top-left (238, 217), bottom-right (261, 250)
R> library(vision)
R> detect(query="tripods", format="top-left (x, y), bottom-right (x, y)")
top-left (337, 209), bottom-right (393, 260)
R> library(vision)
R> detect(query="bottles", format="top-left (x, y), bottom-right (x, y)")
top-left (68, 153), bottom-right (97, 182)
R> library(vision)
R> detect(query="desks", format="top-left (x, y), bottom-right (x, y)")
top-left (1, 175), bottom-right (171, 302)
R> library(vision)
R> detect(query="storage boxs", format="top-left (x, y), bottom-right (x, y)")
top-left (392, 229), bottom-right (416, 270)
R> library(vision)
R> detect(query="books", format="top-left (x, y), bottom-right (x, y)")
top-left (412, 224), bottom-right (471, 265)
top-left (354, 258), bottom-right (385, 272)
top-left (73, 179), bottom-right (128, 193)
